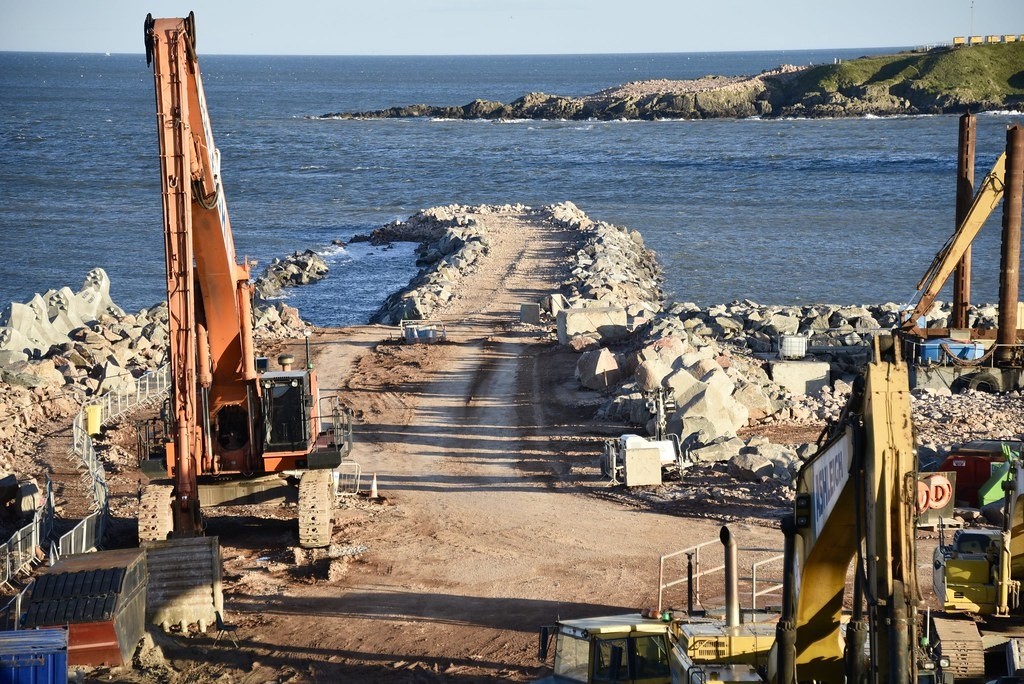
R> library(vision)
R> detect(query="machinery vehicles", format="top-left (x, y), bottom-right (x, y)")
top-left (534, 330), bottom-right (1022, 684)
top-left (876, 110), bottom-right (1024, 398)
top-left (127, 8), bottom-right (357, 634)
